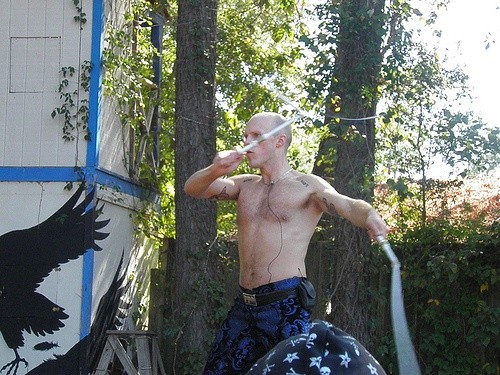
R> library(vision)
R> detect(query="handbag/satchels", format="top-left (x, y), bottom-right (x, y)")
top-left (297, 279), bottom-right (317, 308)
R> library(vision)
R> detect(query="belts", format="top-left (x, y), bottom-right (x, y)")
top-left (237, 288), bottom-right (297, 307)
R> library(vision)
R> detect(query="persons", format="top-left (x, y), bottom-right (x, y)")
top-left (183, 112), bottom-right (391, 375)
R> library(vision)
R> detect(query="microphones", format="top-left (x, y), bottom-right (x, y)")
top-left (270, 180), bottom-right (273, 185)
